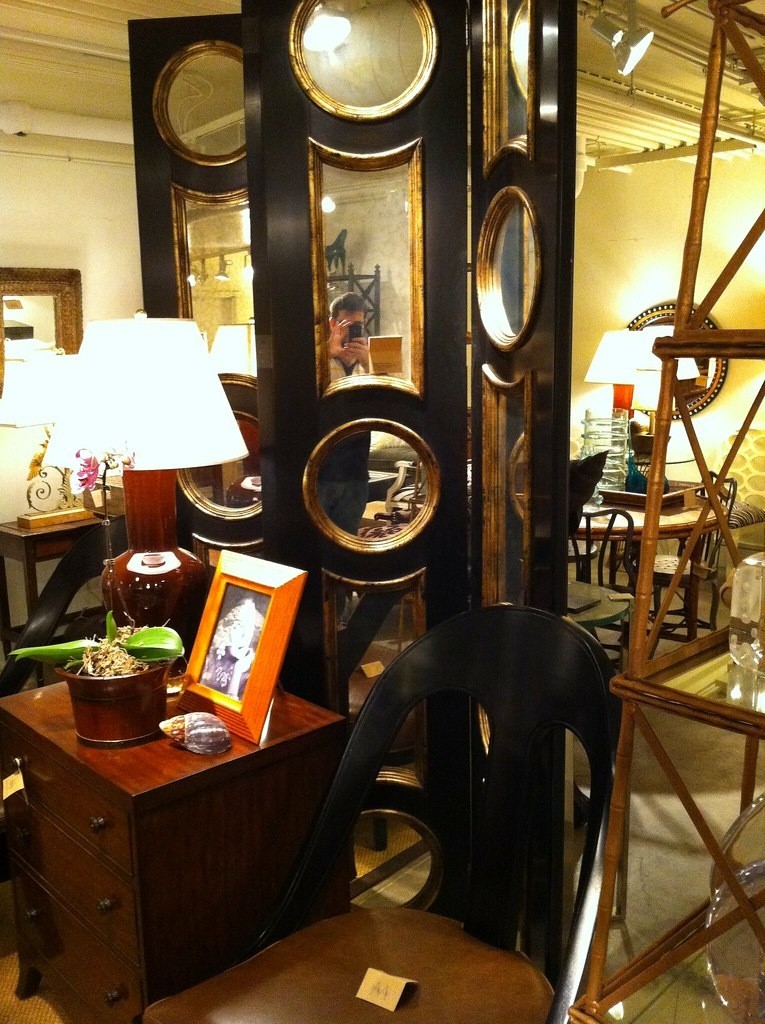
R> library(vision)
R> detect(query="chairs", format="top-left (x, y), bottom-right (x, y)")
top-left (135, 600), bottom-right (620, 1023)
top-left (0, 517), bottom-right (122, 701)
top-left (569, 471), bottom-right (737, 664)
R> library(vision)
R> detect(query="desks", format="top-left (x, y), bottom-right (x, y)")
top-left (574, 503), bottom-right (729, 647)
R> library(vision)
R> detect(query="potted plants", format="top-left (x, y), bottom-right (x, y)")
top-left (0, 608), bottom-right (0, 608)
top-left (6, 607), bottom-right (188, 751)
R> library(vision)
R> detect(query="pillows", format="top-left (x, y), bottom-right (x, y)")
top-left (722, 500), bottom-right (765, 529)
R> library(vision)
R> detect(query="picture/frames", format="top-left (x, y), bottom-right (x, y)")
top-left (173, 543), bottom-right (310, 746)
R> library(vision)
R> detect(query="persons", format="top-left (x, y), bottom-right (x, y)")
top-left (198, 597), bottom-right (265, 701)
top-left (316, 291), bottom-right (372, 537)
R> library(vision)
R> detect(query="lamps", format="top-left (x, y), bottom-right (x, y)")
top-left (583, 332), bottom-right (675, 458)
top-left (209, 324), bottom-right (258, 377)
top-left (214, 254), bottom-right (233, 282)
top-left (38, 309), bottom-right (254, 652)
top-left (188, 258), bottom-right (209, 286)
top-left (589, 0), bottom-right (648, 76)
top-left (642, 324), bottom-right (700, 380)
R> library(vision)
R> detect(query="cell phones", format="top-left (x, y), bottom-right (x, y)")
top-left (349, 324), bottom-right (361, 342)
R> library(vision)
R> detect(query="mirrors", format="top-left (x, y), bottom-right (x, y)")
top-left (133, 1), bottom-right (582, 928)
top-left (627, 304), bottom-right (727, 418)
top-left (0, 265), bottom-right (85, 425)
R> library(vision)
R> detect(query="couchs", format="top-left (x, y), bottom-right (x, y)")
top-left (657, 428), bottom-right (765, 592)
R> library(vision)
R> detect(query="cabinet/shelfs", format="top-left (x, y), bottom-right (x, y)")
top-left (0, 655), bottom-right (347, 1023)
top-left (564, 0), bottom-right (765, 1023)
